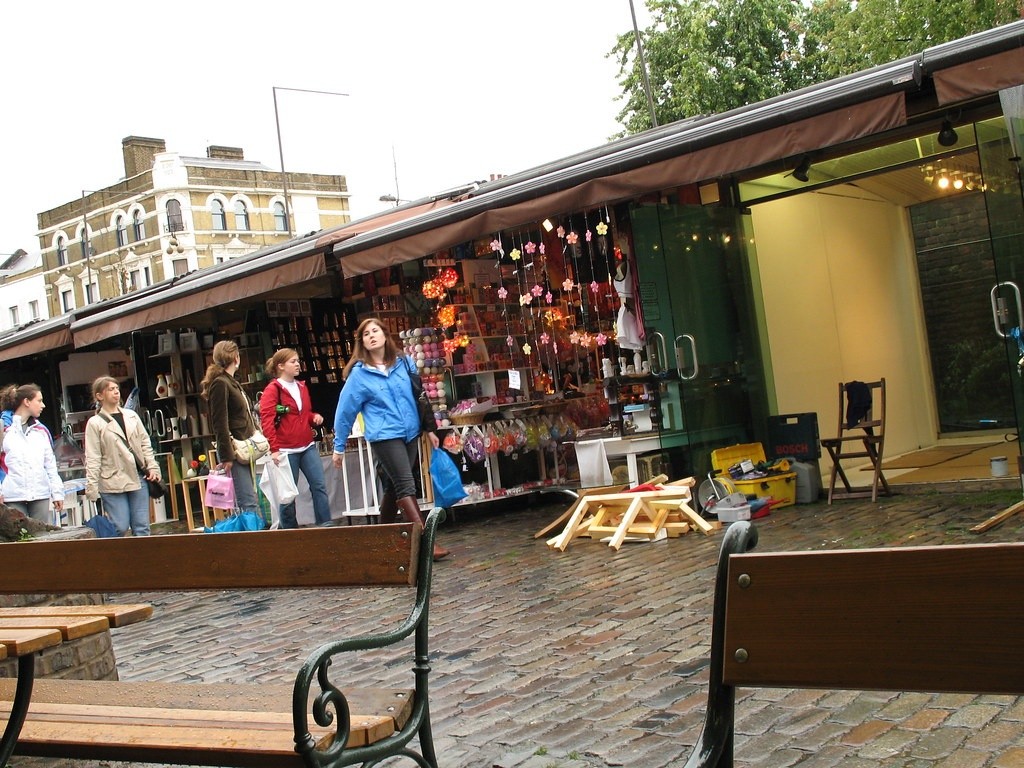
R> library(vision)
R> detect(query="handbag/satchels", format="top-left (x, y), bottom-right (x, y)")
top-left (428, 447), bottom-right (468, 508)
top-left (398, 356), bottom-right (439, 432)
top-left (142, 467), bottom-right (169, 499)
top-left (255, 451), bottom-right (299, 504)
top-left (206, 463), bottom-right (237, 510)
top-left (232, 430), bottom-right (269, 465)
top-left (82, 515), bottom-right (118, 537)
top-left (254, 383), bottom-right (282, 427)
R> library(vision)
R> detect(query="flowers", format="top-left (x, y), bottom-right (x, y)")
top-left (191, 454), bottom-right (207, 469)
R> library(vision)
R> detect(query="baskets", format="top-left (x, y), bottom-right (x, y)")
top-left (500, 405), bottom-right (541, 420)
top-left (448, 412), bottom-right (485, 425)
top-left (541, 400), bottom-right (569, 414)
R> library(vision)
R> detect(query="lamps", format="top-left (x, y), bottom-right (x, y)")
top-left (939, 121), bottom-right (957, 146)
top-left (793, 157), bottom-right (811, 180)
top-left (921, 167), bottom-right (1023, 195)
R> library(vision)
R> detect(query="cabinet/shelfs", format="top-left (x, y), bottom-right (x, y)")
top-left (62, 287), bottom-right (658, 512)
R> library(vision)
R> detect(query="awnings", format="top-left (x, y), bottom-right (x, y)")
top-left (0, 17), bottom-right (1024, 364)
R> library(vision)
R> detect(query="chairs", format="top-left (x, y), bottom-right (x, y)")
top-left (820, 378), bottom-right (886, 506)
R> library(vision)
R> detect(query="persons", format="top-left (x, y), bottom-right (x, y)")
top-left (201, 340), bottom-right (259, 516)
top-left (0, 383), bottom-right (64, 523)
top-left (332, 318), bottom-right (450, 560)
top-left (85, 377), bottom-right (161, 536)
top-left (261, 348), bottom-right (332, 529)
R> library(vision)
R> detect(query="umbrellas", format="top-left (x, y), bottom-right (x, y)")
top-left (255, 403), bottom-right (290, 413)
top-left (204, 469), bottom-right (265, 533)
top-left (85, 502), bottom-right (121, 538)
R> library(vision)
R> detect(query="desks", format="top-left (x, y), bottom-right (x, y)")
top-left (179, 477), bottom-right (232, 531)
top-left (1, 605), bottom-right (156, 766)
top-left (680, 519), bottom-right (1023, 768)
top-left (602, 423), bottom-right (744, 491)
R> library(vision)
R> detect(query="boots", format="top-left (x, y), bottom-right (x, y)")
top-left (376, 512), bottom-right (399, 524)
top-left (395, 496), bottom-right (451, 559)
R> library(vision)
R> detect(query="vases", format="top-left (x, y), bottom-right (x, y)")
top-left (201, 467), bottom-right (208, 475)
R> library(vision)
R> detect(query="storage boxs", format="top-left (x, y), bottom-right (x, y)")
top-left (767, 412), bottom-right (823, 461)
top-left (711, 443), bottom-right (797, 511)
top-left (777, 457), bottom-right (819, 504)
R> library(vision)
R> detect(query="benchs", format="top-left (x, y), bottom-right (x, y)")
top-left (1, 506), bottom-right (447, 767)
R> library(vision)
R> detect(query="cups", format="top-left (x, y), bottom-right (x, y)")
top-left (165, 414), bottom-right (209, 440)
top-left (256, 372), bottom-right (264, 381)
top-left (989, 456), bottom-right (1009, 477)
top-left (166, 375), bottom-right (175, 396)
top-left (248, 374), bottom-right (254, 383)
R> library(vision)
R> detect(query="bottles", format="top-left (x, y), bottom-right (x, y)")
top-left (272, 311), bottom-right (353, 384)
top-left (155, 374), bottom-right (168, 397)
top-left (185, 369), bottom-right (194, 393)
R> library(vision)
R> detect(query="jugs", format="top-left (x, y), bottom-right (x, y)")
top-left (773, 457), bottom-right (818, 504)
